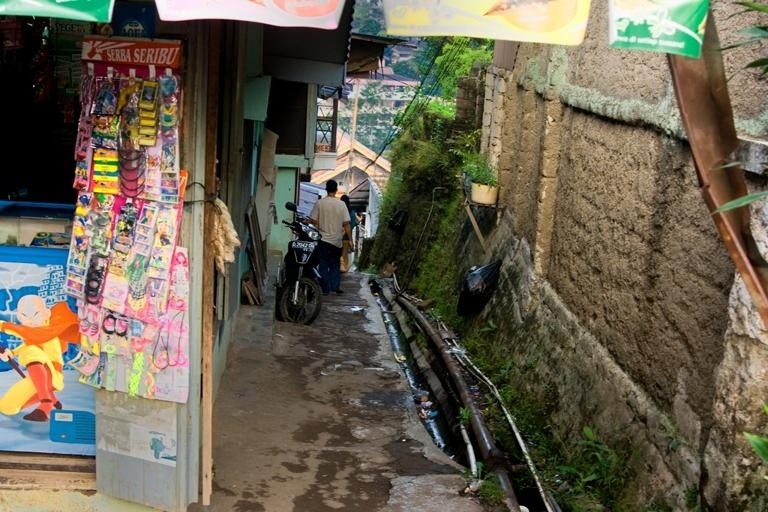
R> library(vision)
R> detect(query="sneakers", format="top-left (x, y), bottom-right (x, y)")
top-left (323, 289), bottom-right (345, 296)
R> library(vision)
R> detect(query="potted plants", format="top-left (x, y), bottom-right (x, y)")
top-left (443, 126), bottom-right (500, 206)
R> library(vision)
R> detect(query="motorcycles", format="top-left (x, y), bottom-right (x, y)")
top-left (276, 202), bottom-right (323, 326)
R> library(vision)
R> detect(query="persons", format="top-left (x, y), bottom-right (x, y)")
top-left (309, 179), bottom-right (362, 297)
